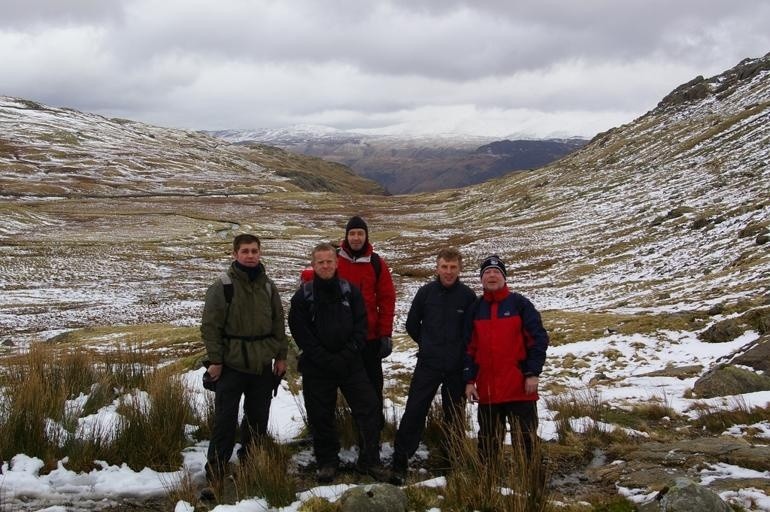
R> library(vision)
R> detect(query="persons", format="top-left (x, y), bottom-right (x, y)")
top-left (200, 234), bottom-right (289, 504)
top-left (460, 256), bottom-right (550, 462)
top-left (288, 243), bottom-right (393, 481)
top-left (392, 246), bottom-right (478, 486)
top-left (300, 216), bottom-right (396, 450)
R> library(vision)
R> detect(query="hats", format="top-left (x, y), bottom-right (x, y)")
top-left (345, 216), bottom-right (369, 255)
top-left (479, 255), bottom-right (507, 279)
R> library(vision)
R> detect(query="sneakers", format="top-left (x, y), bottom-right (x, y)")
top-left (356, 459), bottom-right (393, 479)
top-left (389, 464), bottom-right (408, 488)
top-left (316, 464), bottom-right (338, 484)
